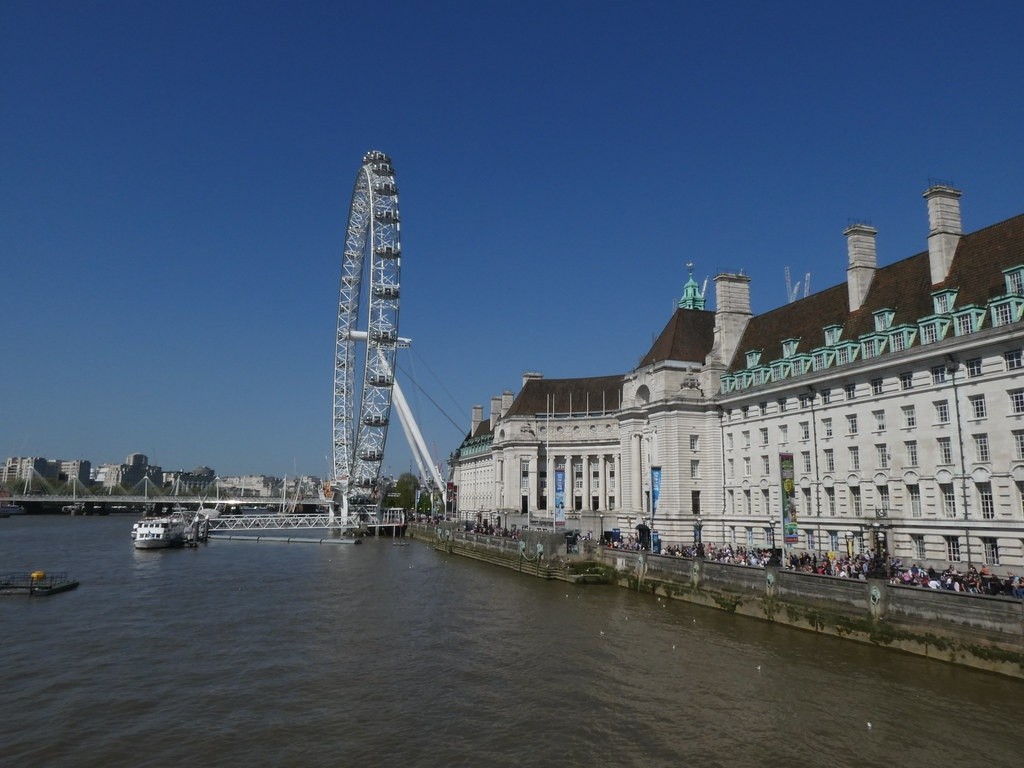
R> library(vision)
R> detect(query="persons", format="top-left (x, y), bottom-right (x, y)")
top-left (657, 541), bottom-right (773, 567)
top-left (471, 522), bottom-right (523, 539)
top-left (405, 510), bottom-right (451, 526)
top-left (787, 547), bottom-right (876, 581)
top-left (601, 533), bottom-right (649, 551)
top-left (887, 555), bottom-right (1024, 598)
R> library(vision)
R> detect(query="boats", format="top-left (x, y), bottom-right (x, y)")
top-left (0, 503), bottom-right (26, 514)
top-left (131, 510), bottom-right (208, 549)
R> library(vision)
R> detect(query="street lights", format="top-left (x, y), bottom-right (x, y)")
top-left (696, 516), bottom-right (706, 558)
top-left (410, 507), bottom-right (469, 532)
top-left (872, 522), bottom-right (880, 579)
top-left (599, 514), bottom-right (606, 545)
top-left (641, 516), bottom-right (649, 552)
top-left (502, 510), bottom-right (509, 538)
top-left (767, 517), bottom-right (782, 567)
